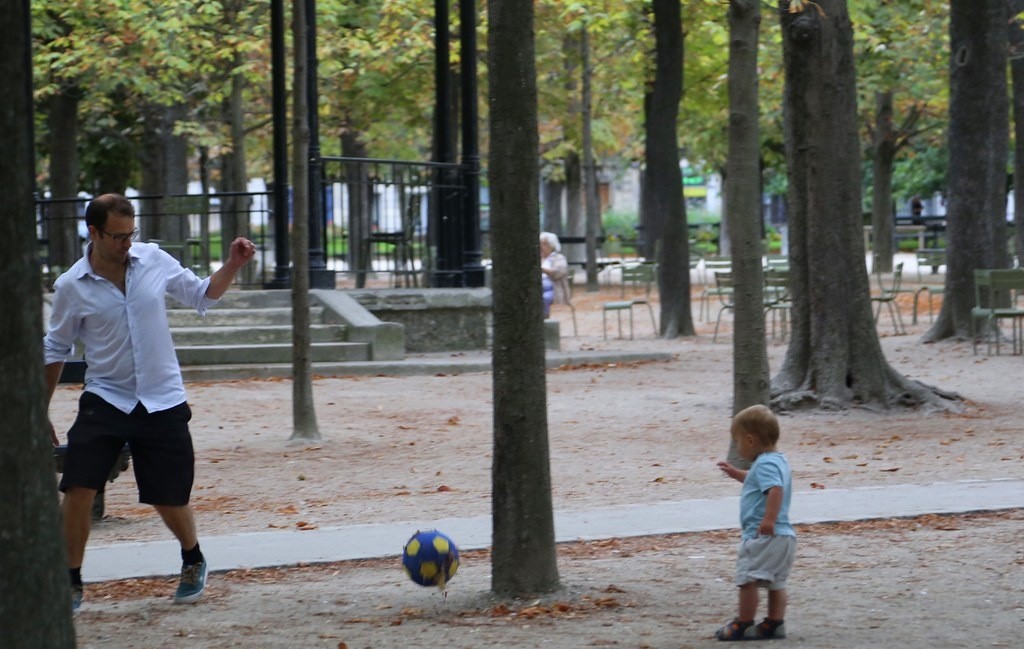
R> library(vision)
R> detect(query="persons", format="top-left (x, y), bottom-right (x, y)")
top-left (716, 404), bottom-right (797, 641)
top-left (540, 231), bottom-right (567, 318)
top-left (44, 193), bottom-right (255, 613)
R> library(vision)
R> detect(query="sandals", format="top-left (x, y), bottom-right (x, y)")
top-left (716, 617), bottom-right (754, 640)
top-left (744, 617), bottom-right (785, 639)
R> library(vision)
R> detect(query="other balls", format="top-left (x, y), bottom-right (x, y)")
top-left (401, 528), bottom-right (462, 590)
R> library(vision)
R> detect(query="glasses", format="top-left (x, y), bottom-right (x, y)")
top-left (104, 227), bottom-right (140, 244)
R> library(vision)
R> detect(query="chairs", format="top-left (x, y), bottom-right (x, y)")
top-left (150, 193), bottom-right (211, 278)
top-left (602, 254), bottom-right (793, 345)
top-left (356, 193), bottom-right (422, 288)
top-left (871, 262), bottom-right (907, 336)
top-left (915, 285), bottom-right (945, 325)
top-left (971, 268), bottom-right (1016, 356)
top-left (550, 268), bottom-right (578, 338)
top-left (987, 267), bottom-right (1024, 356)
top-left (875, 253), bottom-right (916, 325)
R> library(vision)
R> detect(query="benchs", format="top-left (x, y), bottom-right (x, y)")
top-left (916, 248), bottom-right (945, 274)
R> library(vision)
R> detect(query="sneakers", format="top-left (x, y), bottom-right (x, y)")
top-left (173, 552), bottom-right (208, 604)
top-left (71, 578), bottom-right (83, 619)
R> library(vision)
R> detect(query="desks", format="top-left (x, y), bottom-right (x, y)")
top-left (567, 260), bottom-right (621, 273)
